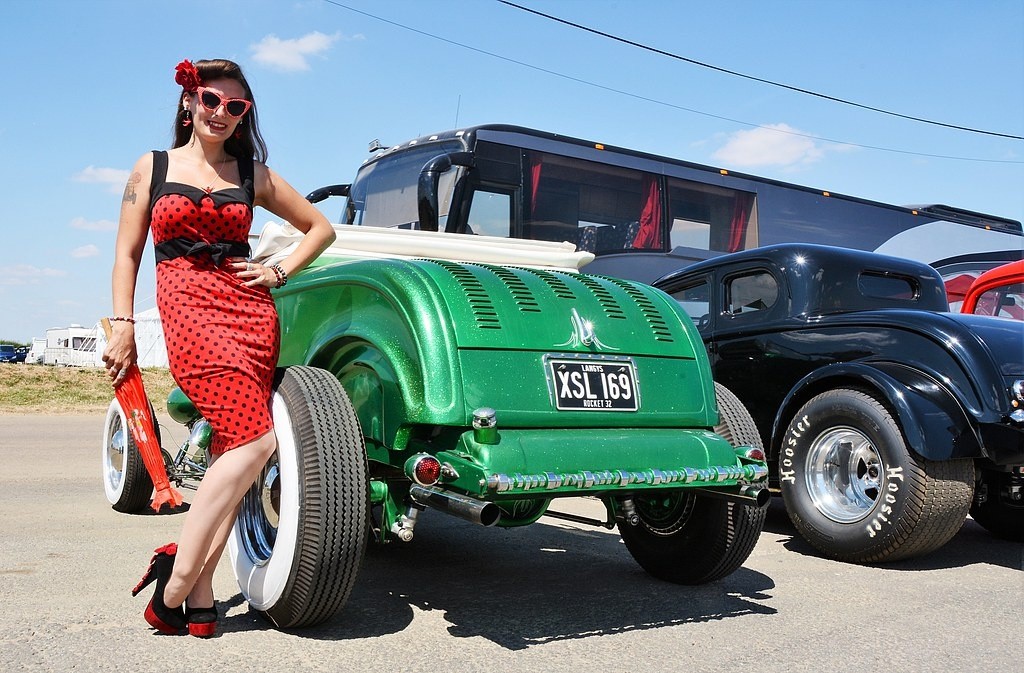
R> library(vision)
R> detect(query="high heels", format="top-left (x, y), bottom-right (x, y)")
top-left (132, 543), bottom-right (185, 635)
top-left (186, 588), bottom-right (217, 636)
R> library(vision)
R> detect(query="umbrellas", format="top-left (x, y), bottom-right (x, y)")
top-left (101, 317), bottom-right (183, 512)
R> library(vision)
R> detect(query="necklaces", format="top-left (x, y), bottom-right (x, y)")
top-left (178, 146), bottom-right (228, 193)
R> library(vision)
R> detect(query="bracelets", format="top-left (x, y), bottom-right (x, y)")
top-left (270, 266), bottom-right (281, 289)
top-left (275, 264), bottom-right (287, 286)
top-left (109, 316), bottom-right (135, 323)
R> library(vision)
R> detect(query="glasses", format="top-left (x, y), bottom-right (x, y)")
top-left (192, 86), bottom-right (252, 119)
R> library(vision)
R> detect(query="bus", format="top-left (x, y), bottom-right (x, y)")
top-left (301, 123), bottom-right (1023, 337)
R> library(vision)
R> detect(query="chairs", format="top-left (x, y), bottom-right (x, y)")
top-left (544, 222), bottom-right (641, 254)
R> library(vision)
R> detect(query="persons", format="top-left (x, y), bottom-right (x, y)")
top-left (99, 58), bottom-right (336, 638)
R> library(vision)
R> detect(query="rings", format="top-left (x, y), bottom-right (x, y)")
top-left (245, 256), bottom-right (252, 263)
top-left (246, 262), bottom-right (252, 271)
top-left (118, 368), bottom-right (129, 377)
top-left (111, 365), bottom-right (118, 373)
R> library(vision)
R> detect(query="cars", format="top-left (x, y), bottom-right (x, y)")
top-left (0, 344), bottom-right (28, 365)
top-left (960, 261), bottom-right (1023, 322)
top-left (101, 223), bottom-right (778, 631)
top-left (646, 243), bottom-right (1024, 564)
